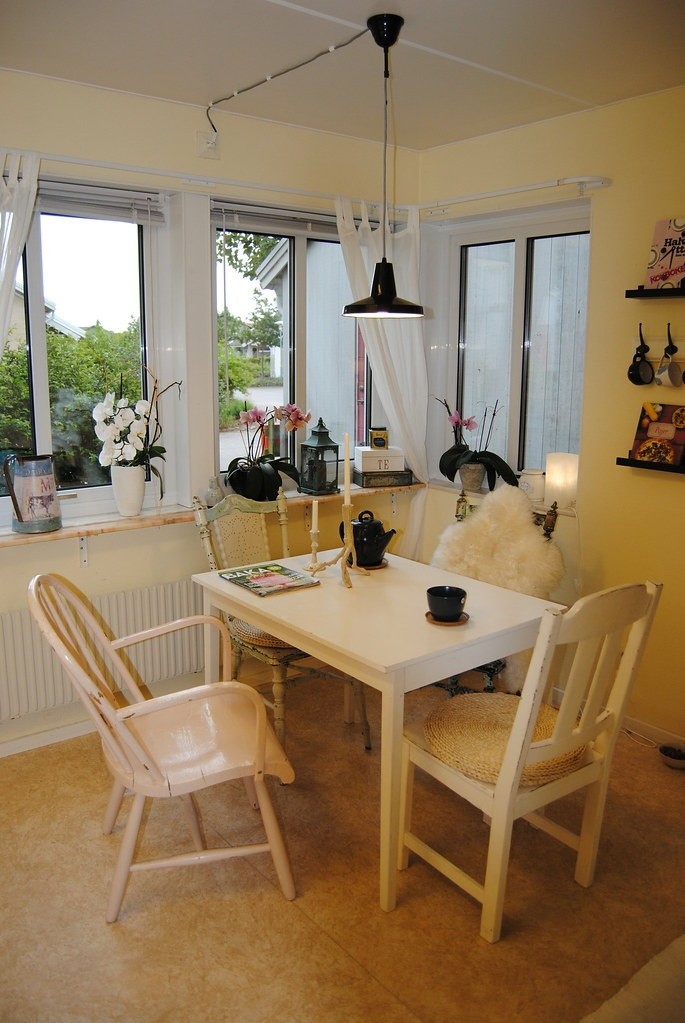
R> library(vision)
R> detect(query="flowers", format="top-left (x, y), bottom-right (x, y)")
top-left (93, 365), bottom-right (182, 501)
top-left (224, 400), bottom-right (312, 489)
top-left (436, 397), bottom-right (501, 451)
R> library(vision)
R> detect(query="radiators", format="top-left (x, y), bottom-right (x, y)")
top-left (1, 581), bottom-right (204, 723)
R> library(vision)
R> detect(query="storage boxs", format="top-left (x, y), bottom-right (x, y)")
top-left (355, 446), bottom-right (405, 472)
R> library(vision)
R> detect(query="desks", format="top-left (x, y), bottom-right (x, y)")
top-left (191, 546), bottom-right (568, 912)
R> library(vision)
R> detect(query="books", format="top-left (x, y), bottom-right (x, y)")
top-left (645, 219), bottom-right (685, 289)
top-left (630, 403), bottom-right (685, 465)
top-left (218, 563), bottom-right (320, 597)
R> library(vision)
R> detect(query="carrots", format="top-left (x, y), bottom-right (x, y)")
top-left (643, 402), bottom-right (659, 421)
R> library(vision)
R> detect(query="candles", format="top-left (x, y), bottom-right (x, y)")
top-left (343, 433), bottom-right (352, 504)
top-left (311, 500), bottom-right (319, 533)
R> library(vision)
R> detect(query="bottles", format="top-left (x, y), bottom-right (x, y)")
top-left (518, 468), bottom-right (546, 503)
top-left (369, 426), bottom-right (388, 450)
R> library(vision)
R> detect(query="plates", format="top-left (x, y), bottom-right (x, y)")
top-left (425, 611), bottom-right (471, 626)
top-left (345, 558), bottom-right (389, 570)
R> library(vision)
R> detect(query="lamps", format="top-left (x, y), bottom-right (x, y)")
top-left (343, 14), bottom-right (424, 317)
top-left (544, 452), bottom-right (580, 508)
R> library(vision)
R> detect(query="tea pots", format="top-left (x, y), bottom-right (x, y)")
top-left (339, 510), bottom-right (397, 565)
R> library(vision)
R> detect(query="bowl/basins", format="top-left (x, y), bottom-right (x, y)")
top-left (658, 741), bottom-right (685, 768)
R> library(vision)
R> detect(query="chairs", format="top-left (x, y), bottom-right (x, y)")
top-left (398, 579), bottom-right (663, 944)
top-left (194, 486), bottom-right (371, 749)
top-left (27, 571), bottom-right (296, 923)
top-left (430, 489), bottom-right (558, 698)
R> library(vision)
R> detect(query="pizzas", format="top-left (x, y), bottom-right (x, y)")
top-left (672, 407), bottom-right (685, 428)
top-left (635, 438), bottom-right (675, 465)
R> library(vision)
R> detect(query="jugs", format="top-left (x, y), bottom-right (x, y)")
top-left (3, 453), bottom-right (62, 532)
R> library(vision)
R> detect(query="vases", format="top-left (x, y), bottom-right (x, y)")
top-left (230, 467), bottom-right (279, 501)
top-left (110, 465), bottom-right (145, 517)
top-left (458, 464), bottom-right (484, 491)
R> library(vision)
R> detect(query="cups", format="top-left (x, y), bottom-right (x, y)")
top-left (544, 453), bottom-right (579, 510)
top-left (627, 352), bottom-right (653, 385)
top-left (426, 585), bottom-right (467, 621)
top-left (654, 353), bottom-right (683, 388)
